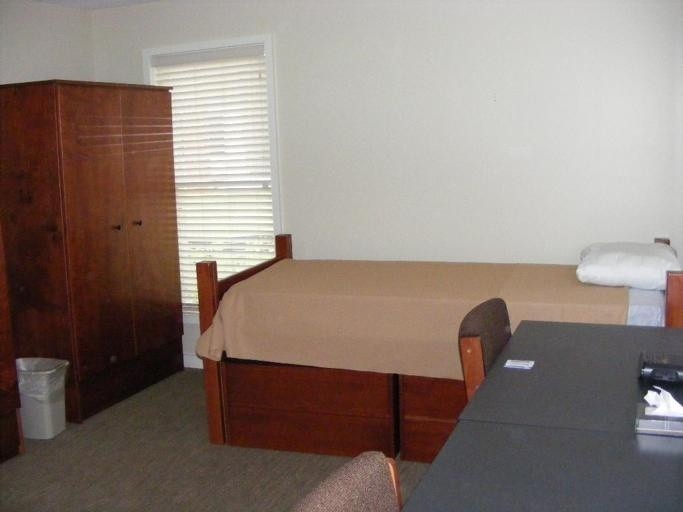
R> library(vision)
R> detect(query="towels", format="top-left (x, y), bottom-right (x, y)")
top-left (16, 358), bottom-right (70, 439)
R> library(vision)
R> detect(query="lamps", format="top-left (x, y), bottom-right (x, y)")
top-left (288, 450), bottom-right (402, 512)
top-left (457, 299), bottom-right (511, 399)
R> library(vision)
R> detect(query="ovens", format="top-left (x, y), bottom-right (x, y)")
top-left (195, 233), bottom-right (683, 465)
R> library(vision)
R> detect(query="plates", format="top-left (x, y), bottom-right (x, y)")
top-left (0, 78), bottom-right (185, 423)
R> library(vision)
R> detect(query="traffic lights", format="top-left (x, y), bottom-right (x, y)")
top-left (576, 241), bottom-right (683, 290)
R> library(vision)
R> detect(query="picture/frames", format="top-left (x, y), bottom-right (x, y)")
top-left (395, 318), bottom-right (683, 512)
